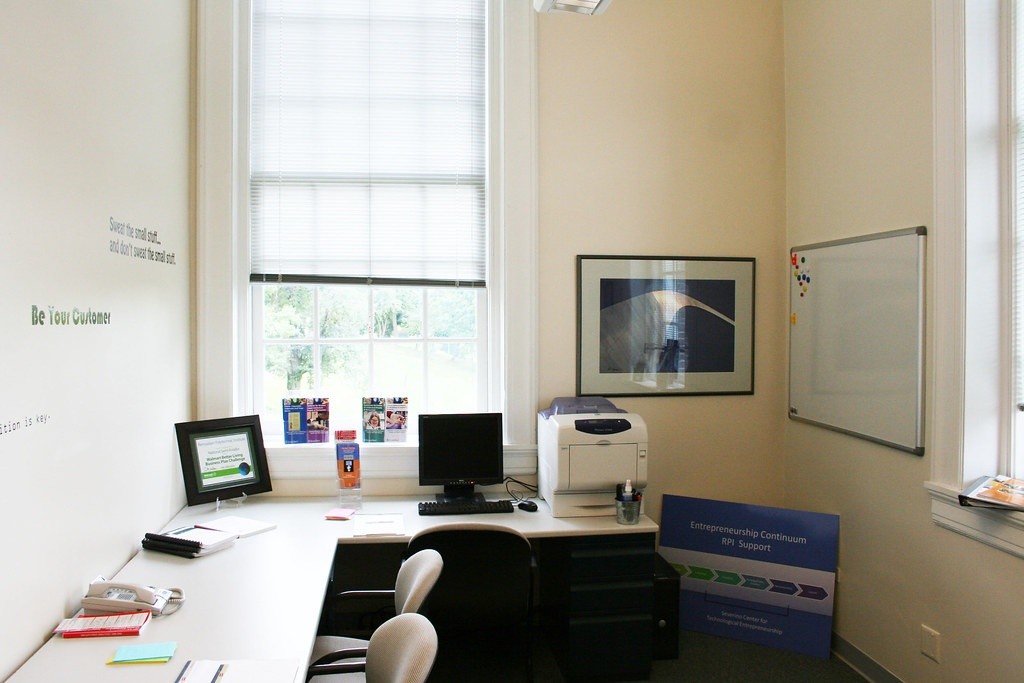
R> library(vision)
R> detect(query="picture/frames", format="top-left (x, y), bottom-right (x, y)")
top-left (573, 251), bottom-right (757, 399)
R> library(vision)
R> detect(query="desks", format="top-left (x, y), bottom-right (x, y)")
top-left (1, 492), bottom-right (662, 683)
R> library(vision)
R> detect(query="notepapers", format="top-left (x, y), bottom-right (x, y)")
top-left (105, 651), bottom-right (171, 665)
top-left (113, 641), bottom-right (176, 663)
top-left (325, 508), bottom-right (357, 520)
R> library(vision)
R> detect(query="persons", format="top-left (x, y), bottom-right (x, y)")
top-left (305, 397), bottom-right (406, 434)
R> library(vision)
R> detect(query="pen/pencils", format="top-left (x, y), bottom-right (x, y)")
top-left (616, 479), bottom-right (642, 521)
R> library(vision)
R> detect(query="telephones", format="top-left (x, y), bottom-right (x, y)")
top-left (80, 574), bottom-right (173, 616)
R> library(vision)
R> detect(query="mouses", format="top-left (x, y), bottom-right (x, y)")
top-left (518, 501), bottom-right (538, 512)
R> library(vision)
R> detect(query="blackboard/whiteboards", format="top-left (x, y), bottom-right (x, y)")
top-left (787, 226), bottom-right (928, 457)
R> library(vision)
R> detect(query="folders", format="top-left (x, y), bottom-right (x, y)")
top-left (958, 475), bottom-right (1024, 512)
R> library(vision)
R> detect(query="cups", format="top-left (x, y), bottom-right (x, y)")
top-left (612, 495), bottom-right (643, 525)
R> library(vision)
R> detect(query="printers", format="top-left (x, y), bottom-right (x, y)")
top-left (537, 396), bottom-right (648, 517)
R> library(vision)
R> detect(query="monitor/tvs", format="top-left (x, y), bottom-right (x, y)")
top-left (419, 412), bottom-right (504, 486)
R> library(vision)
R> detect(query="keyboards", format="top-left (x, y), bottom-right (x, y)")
top-left (418, 500), bottom-right (513, 515)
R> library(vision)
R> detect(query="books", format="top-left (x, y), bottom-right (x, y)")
top-left (194, 515), bottom-right (279, 539)
top-left (142, 526), bottom-right (238, 559)
top-left (50, 610), bottom-right (152, 639)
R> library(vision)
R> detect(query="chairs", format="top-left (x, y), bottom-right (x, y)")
top-left (406, 522), bottom-right (539, 683)
top-left (308, 546), bottom-right (441, 671)
top-left (306, 613), bottom-right (441, 683)
